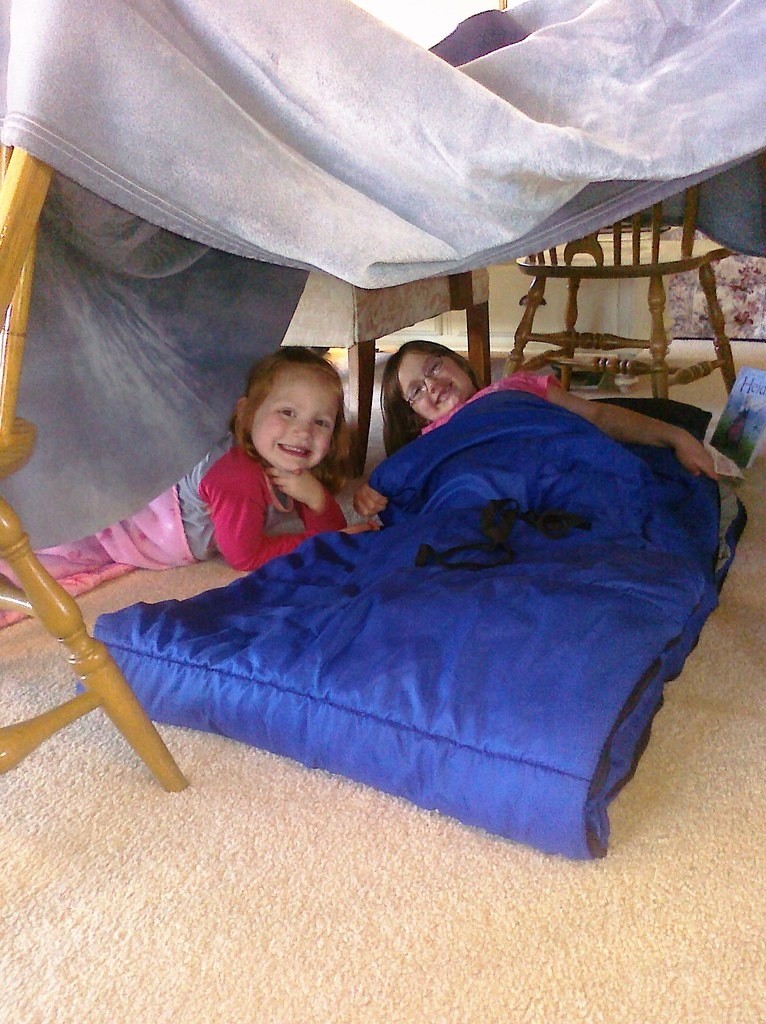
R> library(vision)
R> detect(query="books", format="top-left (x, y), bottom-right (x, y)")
top-left (563, 371), bottom-right (604, 389)
top-left (710, 358), bottom-right (766, 469)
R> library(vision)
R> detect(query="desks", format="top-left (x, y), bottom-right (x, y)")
top-left (281, 269), bottom-right (491, 478)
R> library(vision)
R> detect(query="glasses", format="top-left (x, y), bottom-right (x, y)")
top-left (406, 349), bottom-right (443, 405)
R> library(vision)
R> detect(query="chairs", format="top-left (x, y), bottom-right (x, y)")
top-left (503, 182), bottom-right (739, 401)
top-left (0, 0), bottom-right (187, 793)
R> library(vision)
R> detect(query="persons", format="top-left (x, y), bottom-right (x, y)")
top-left (353, 340), bottom-right (718, 555)
top-left (72, 345), bottom-right (382, 574)
